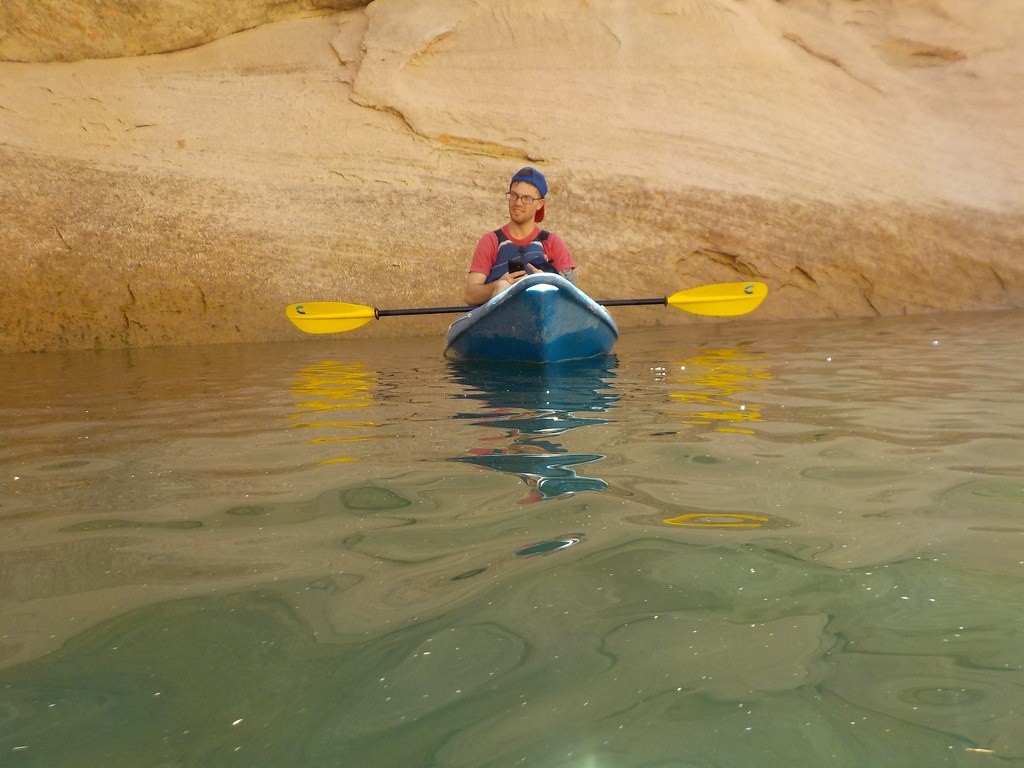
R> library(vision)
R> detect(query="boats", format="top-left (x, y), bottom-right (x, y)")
top-left (443, 269), bottom-right (621, 366)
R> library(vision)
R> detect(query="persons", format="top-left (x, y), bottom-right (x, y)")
top-left (463, 167), bottom-right (574, 305)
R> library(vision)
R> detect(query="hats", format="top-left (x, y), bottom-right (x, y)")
top-left (510, 167), bottom-right (547, 222)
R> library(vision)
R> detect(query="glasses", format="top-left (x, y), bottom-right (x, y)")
top-left (506, 191), bottom-right (543, 204)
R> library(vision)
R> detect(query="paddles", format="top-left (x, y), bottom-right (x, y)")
top-left (285, 281), bottom-right (769, 335)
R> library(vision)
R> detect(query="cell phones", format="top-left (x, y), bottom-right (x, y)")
top-left (507, 260), bottom-right (526, 279)
